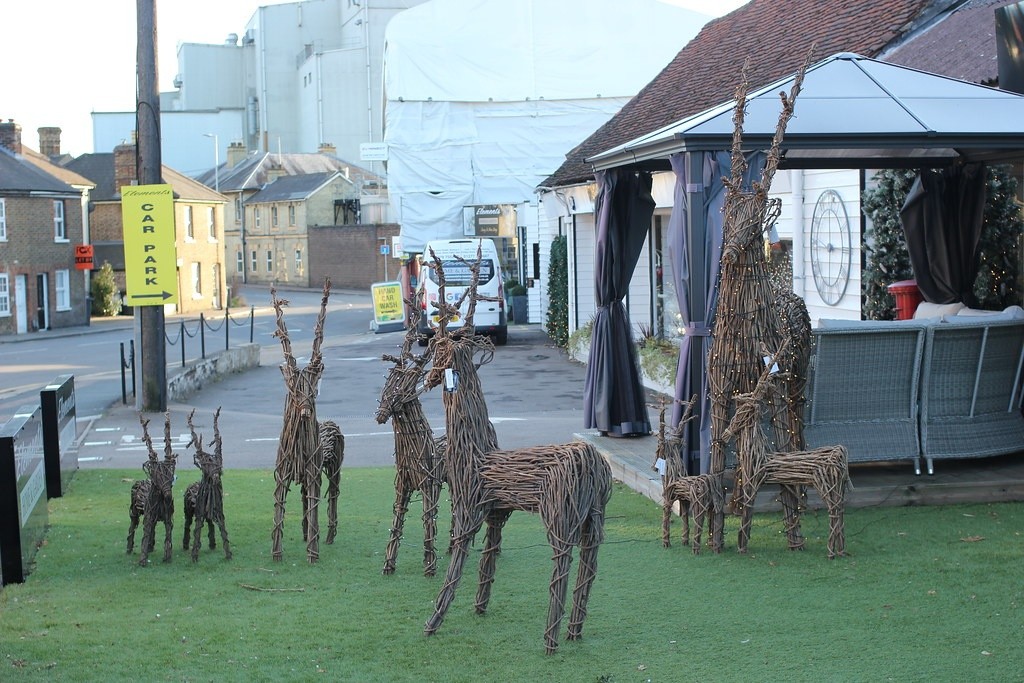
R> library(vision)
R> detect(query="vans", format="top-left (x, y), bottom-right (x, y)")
top-left (412, 238), bottom-right (509, 346)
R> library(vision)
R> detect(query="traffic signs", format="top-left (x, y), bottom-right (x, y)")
top-left (119, 186), bottom-right (179, 307)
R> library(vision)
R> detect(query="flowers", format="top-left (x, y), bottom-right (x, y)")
top-left (670, 311), bottom-right (686, 338)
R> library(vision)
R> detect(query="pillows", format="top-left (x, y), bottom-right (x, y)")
top-left (957, 305), bottom-right (1003, 315)
top-left (818, 316), bottom-right (942, 327)
top-left (915, 300), bottom-right (964, 320)
top-left (944, 307), bottom-right (1017, 323)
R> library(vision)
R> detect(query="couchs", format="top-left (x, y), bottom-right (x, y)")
top-left (801, 302), bottom-right (1024, 475)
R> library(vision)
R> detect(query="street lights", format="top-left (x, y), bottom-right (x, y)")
top-left (203, 132), bottom-right (218, 191)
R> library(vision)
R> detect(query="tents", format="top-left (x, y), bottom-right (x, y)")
top-left (583, 51), bottom-right (1024, 474)
